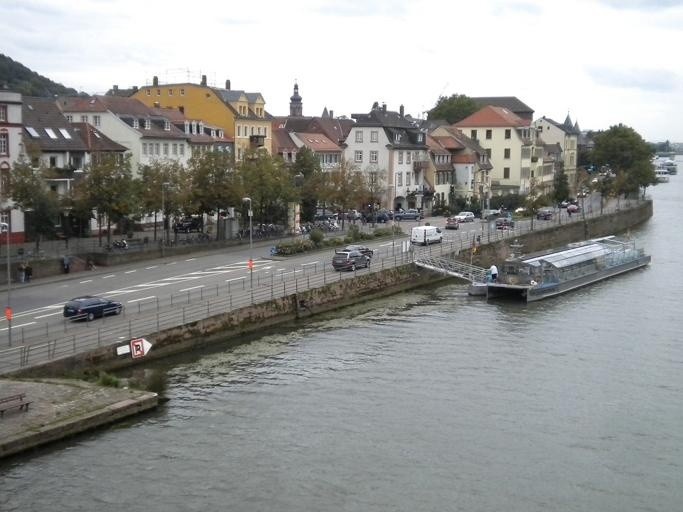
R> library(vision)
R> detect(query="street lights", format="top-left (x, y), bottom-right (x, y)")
top-left (0, 223), bottom-right (12, 348)
top-left (243, 197), bottom-right (253, 288)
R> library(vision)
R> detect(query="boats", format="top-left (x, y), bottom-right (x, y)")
top-left (486, 235), bottom-right (651, 304)
top-left (653, 160), bottom-right (677, 183)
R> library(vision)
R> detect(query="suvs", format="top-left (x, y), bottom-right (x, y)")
top-left (536, 208), bottom-right (552, 220)
top-left (172, 217), bottom-right (202, 233)
top-left (332, 245), bottom-right (373, 271)
top-left (361, 208), bottom-right (422, 223)
top-left (445, 212), bottom-right (475, 230)
top-left (496, 217), bottom-right (514, 229)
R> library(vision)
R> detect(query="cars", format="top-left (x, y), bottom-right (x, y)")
top-left (63, 296), bottom-right (122, 321)
top-left (558, 177), bottom-right (599, 213)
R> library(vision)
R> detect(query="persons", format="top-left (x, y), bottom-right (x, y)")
top-left (486, 262), bottom-right (498, 283)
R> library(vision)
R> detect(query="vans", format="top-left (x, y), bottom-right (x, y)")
top-left (411, 226), bottom-right (442, 245)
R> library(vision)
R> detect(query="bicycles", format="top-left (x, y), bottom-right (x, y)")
top-left (301, 217), bottom-right (334, 233)
top-left (256, 225), bottom-right (270, 239)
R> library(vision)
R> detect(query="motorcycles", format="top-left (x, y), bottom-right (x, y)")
top-left (113, 239), bottom-right (128, 250)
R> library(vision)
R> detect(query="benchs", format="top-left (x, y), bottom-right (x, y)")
top-left (0, 392), bottom-right (33, 419)
